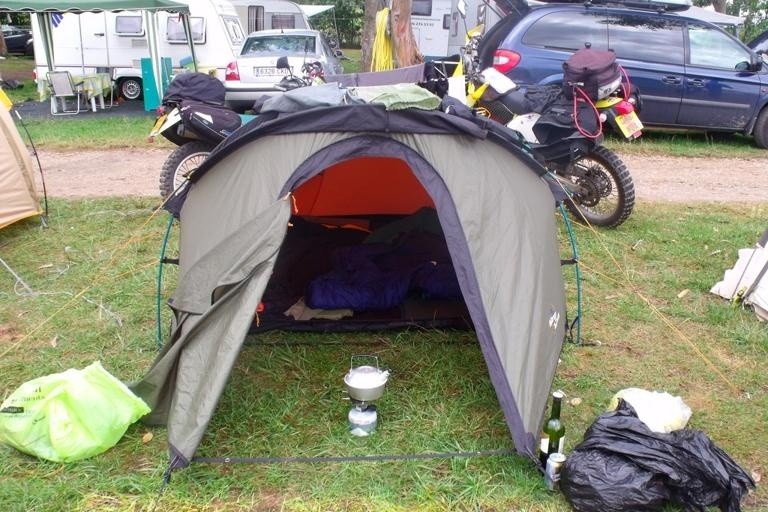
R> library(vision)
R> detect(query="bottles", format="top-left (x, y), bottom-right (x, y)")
top-left (536, 390), bottom-right (567, 476)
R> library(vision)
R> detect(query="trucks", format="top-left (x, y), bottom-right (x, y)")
top-left (28, 0), bottom-right (316, 103)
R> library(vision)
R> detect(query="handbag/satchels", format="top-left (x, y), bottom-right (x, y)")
top-left (164, 71), bottom-right (227, 103)
top-left (560, 48), bottom-right (624, 101)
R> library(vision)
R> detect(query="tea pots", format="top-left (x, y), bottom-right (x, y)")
top-left (342, 352), bottom-right (390, 402)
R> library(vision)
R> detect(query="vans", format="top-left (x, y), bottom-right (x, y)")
top-left (476, 2), bottom-right (768, 151)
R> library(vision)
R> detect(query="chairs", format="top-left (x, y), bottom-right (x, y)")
top-left (42, 71), bottom-right (118, 115)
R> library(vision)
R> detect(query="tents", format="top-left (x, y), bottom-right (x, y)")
top-left (129, 62), bottom-right (583, 495)
top-left (1, 81), bottom-right (50, 234)
top-left (705, 224), bottom-right (768, 330)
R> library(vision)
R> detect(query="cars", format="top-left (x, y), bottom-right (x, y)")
top-left (2, 28), bottom-right (34, 51)
top-left (225, 27), bottom-right (347, 113)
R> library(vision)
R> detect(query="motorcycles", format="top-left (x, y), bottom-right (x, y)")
top-left (145, 53), bottom-right (326, 229)
top-left (421, 0), bottom-right (644, 228)
